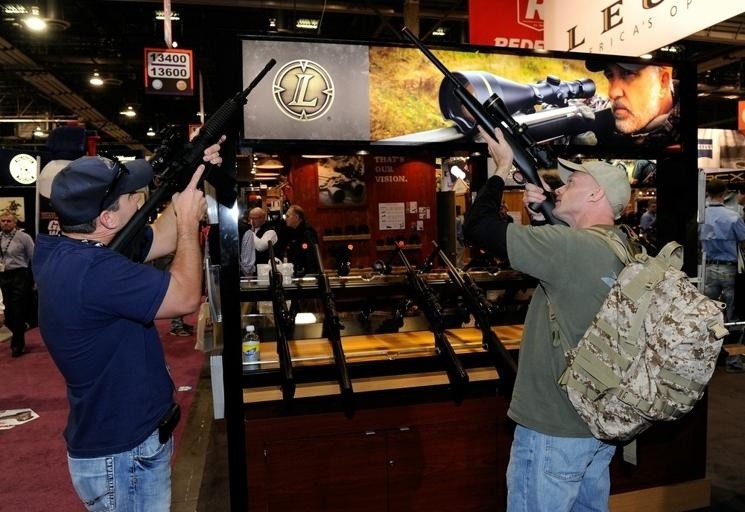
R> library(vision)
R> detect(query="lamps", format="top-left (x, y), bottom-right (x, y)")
top-left (124, 105), bottom-right (136, 116)
top-left (34, 126), bottom-right (42, 135)
top-left (148, 128), bottom-right (155, 136)
top-left (253, 177), bottom-right (276, 181)
top-left (256, 155), bottom-right (283, 169)
top-left (254, 171), bottom-right (280, 176)
top-left (452, 166), bottom-right (466, 181)
top-left (21, 6), bottom-right (49, 33)
top-left (89, 70), bottom-right (104, 86)
top-left (298, 18), bottom-right (317, 28)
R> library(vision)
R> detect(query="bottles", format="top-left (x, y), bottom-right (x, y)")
top-left (242, 324), bottom-right (261, 366)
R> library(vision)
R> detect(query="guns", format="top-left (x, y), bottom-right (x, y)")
top-left (268, 240), bottom-right (296, 399)
top-left (402, 25), bottom-right (570, 229)
top-left (314, 244), bottom-right (356, 418)
top-left (432, 240), bottom-right (518, 384)
top-left (380, 70), bottom-right (614, 144)
top-left (394, 241), bottom-right (469, 405)
top-left (109, 58), bottom-right (276, 252)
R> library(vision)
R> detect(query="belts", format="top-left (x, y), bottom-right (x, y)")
top-left (705, 259), bottom-right (733, 265)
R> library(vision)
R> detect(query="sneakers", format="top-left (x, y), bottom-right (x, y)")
top-left (169, 327), bottom-right (192, 337)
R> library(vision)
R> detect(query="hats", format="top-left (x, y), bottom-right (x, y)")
top-left (556, 156), bottom-right (632, 220)
top-left (584, 59), bottom-right (648, 72)
top-left (50, 155), bottom-right (155, 226)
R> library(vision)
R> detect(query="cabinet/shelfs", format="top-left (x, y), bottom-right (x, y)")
top-left (221, 242), bottom-right (713, 512)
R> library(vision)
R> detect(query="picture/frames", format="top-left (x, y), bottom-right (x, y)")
top-left (0, 196), bottom-right (24, 222)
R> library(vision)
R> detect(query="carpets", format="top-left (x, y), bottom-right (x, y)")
top-left (0, 283), bottom-right (206, 512)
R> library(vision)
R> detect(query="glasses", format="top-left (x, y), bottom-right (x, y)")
top-left (93, 150), bottom-right (131, 214)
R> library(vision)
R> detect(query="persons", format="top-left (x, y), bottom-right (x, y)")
top-left (250, 207), bottom-right (283, 265)
top-left (0, 212), bottom-right (36, 357)
top-left (640, 201), bottom-right (658, 234)
top-left (239, 219), bottom-right (257, 279)
top-left (700, 178), bottom-right (745, 322)
top-left (584, 55), bottom-right (683, 147)
top-left (286, 203), bottom-right (318, 272)
top-left (32, 135), bottom-right (229, 512)
top-left (167, 317), bottom-right (195, 337)
top-left (466, 122), bottom-right (639, 512)
top-left (734, 185), bottom-right (745, 218)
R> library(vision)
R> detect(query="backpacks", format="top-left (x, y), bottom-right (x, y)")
top-left (542, 227), bottom-right (730, 444)
